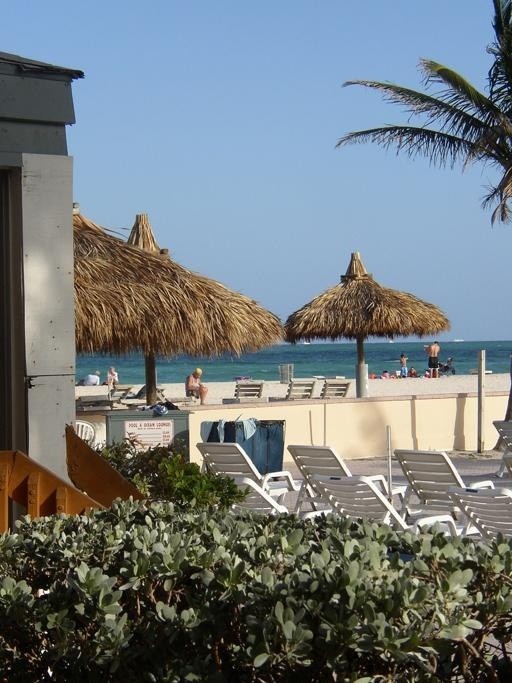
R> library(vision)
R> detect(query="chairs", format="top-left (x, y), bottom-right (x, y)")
top-left (287, 444), bottom-right (423, 519)
top-left (492, 421), bottom-right (511, 478)
top-left (109, 385), bottom-right (148, 409)
top-left (222, 378), bottom-right (353, 406)
top-left (446, 485), bottom-right (511, 542)
top-left (394, 449), bottom-right (496, 521)
top-left (196, 442), bottom-right (313, 515)
top-left (312, 474), bottom-right (480, 536)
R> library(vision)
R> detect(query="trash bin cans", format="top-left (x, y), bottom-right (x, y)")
top-left (202, 419), bottom-right (284, 475)
top-left (278, 364), bottom-right (294, 384)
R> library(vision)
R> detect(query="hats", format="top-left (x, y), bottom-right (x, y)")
top-left (194, 368), bottom-right (202, 374)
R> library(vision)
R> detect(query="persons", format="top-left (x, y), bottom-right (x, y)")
top-left (426, 341), bottom-right (440, 378)
top-left (75, 371), bottom-right (100, 385)
top-left (380, 355), bottom-right (417, 379)
top-left (108, 366), bottom-right (118, 392)
top-left (186, 368), bottom-right (208, 405)
